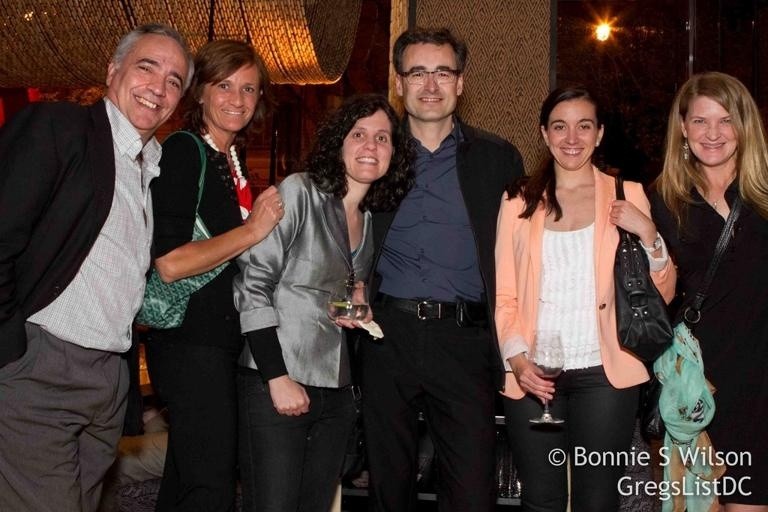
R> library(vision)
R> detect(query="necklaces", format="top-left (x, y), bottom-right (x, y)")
top-left (713, 201), bottom-right (717, 209)
top-left (204, 134), bottom-right (243, 178)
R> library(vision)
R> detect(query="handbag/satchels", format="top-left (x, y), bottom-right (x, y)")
top-left (341, 414), bottom-right (435, 489)
top-left (131, 214), bottom-right (228, 330)
top-left (613, 239), bottom-right (675, 362)
top-left (652, 324), bottom-right (718, 442)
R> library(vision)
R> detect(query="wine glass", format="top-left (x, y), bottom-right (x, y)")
top-left (528, 334), bottom-right (566, 425)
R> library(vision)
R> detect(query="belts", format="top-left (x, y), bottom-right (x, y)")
top-left (375, 290), bottom-right (486, 324)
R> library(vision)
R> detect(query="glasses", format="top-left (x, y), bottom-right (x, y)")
top-left (398, 69), bottom-right (461, 87)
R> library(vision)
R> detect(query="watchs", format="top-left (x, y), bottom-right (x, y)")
top-left (646, 238), bottom-right (662, 252)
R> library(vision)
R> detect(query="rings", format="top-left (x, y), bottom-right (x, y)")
top-left (277, 201), bottom-right (282, 208)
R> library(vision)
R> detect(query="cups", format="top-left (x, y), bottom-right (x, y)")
top-left (324, 282), bottom-right (375, 324)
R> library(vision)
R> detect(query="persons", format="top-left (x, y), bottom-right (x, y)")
top-left (233, 93), bottom-right (419, 512)
top-left (0, 23), bottom-right (196, 512)
top-left (644, 71), bottom-right (768, 512)
top-left (144, 38), bottom-right (283, 512)
top-left (494, 85), bottom-right (677, 512)
top-left (358, 25), bottom-right (525, 512)
